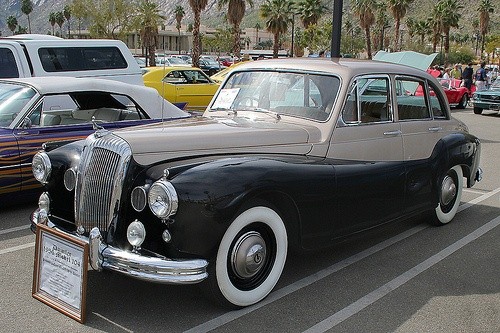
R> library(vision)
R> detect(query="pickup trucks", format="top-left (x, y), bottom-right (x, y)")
top-left (2, 34), bottom-right (146, 110)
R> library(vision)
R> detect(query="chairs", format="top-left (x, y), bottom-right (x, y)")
top-left (29, 107), bottom-right (122, 126)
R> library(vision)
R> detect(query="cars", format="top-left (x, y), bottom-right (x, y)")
top-left (30, 50), bottom-right (482, 310)
top-left (1, 77), bottom-right (191, 195)
top-left (414, 64), bottom-right (500, 114)
top-left (131, 54), bottom-right (353, 109)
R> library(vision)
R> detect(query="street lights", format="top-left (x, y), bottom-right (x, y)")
top-left (291, 12), bottom-right (303, 56)
top-left (381, 25), bottom-right (391, 49)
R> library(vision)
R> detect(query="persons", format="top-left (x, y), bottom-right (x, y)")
top-left (257, 54), bottom-right (266, 60)
top-left (359, 48), bottom-right (369, 59)
top-left (426, 62), bottom-right (492, 103)
top-left (319, 51), bottom-right (324, 57)
top-left (242, 54), bottom-right (251, 62)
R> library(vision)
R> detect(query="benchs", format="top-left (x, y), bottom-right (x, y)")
top-left (325, 100), bottom-right (441, 120)
top-left (274, 106), bottom-right (328, 121)
